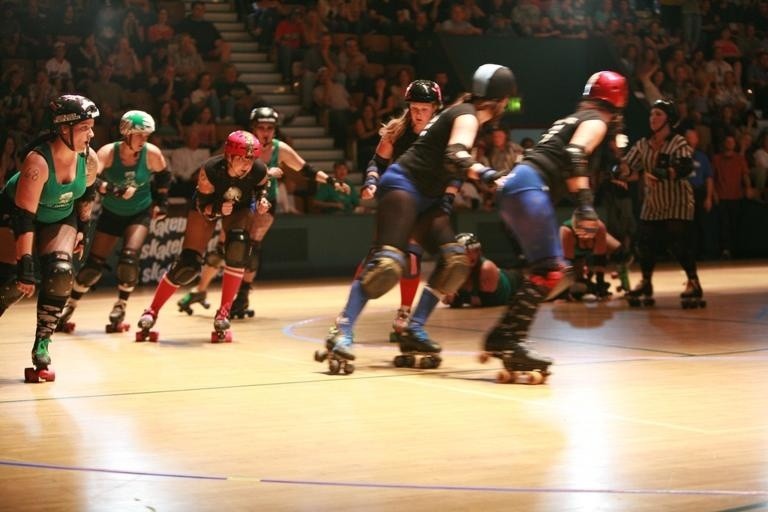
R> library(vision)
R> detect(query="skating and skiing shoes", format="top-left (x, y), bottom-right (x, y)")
top-left (106, 298), bottom-right (131, 333)
top-left (313, 336), bottom-right (357, 375)
top-left (680, 278), bottom-right (707, 311)
top-left (177, 286), bottom-right (211, 316)
top-left (229, 279), bottom-right (255, 320)
top-left (25, 336), bottom-right (56, 385)
top-left (389, 311), bottom-right (410, 341)
top-left (54, 303), bottom-right (77, 332)
top-left (479, 326), bottom-right (552, 386)
top-left (394, 327), bottom-right (442, 371)
top-left (135, 307), bottom-right (159, 343)
top-left (211, 309), bottom-right (232, 344)
top-left (626, 280), bottom-right (656, 308)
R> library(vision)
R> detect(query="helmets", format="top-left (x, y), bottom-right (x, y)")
top-left (472, 62), bottom-right (520, 101)
top-left (654, 99), bottom-right (682, 126)
top-left (583, 71), bottom-right (628, 108)
top-left (403, 80), bottom-right (442, 104)
top-left (248, 106), bottom-right (280, 127)
top-left (47, 94), bottom-right (100, 122)
top-left (119, 110), bottom-right (156, 135)
top-left (225, 130), bottom-right (261, 160)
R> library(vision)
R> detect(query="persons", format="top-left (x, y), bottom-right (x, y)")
top-left (0, 93), bottom-right (101, 368)
top-left (58, 110), bottom-right (175, 324)
top-left (325, 60), bottom-right (520, 361)
top-left (361, 77), bottom-right (468, 333)
top-left (472, 68), bottom-right (632, 370)
top-left (555, 204), bottom-right (635, 297)
top-left (439, 229), bottom-right (610, 307)
top-left (612, 97), bottom-right (705, 300)
top-left (136, 128), bottom-right (274, 331)
top-left (160, 107), bottom-right (352, 315)
top-left (0, 1), bottom-right (768, 264)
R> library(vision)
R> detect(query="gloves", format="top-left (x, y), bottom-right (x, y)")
top-left (478, 166), bottom-right (512, 191)
top-left (16, 253), bottom-right (36, 286)
top-left (154, 192), bottom-right (170, 221)
top-left (76, 217), bottom-right (91, 247)
top-left (573, 188), bottom-right (598, 239)
top-left (326, 176), bottom-right (347, 193)
